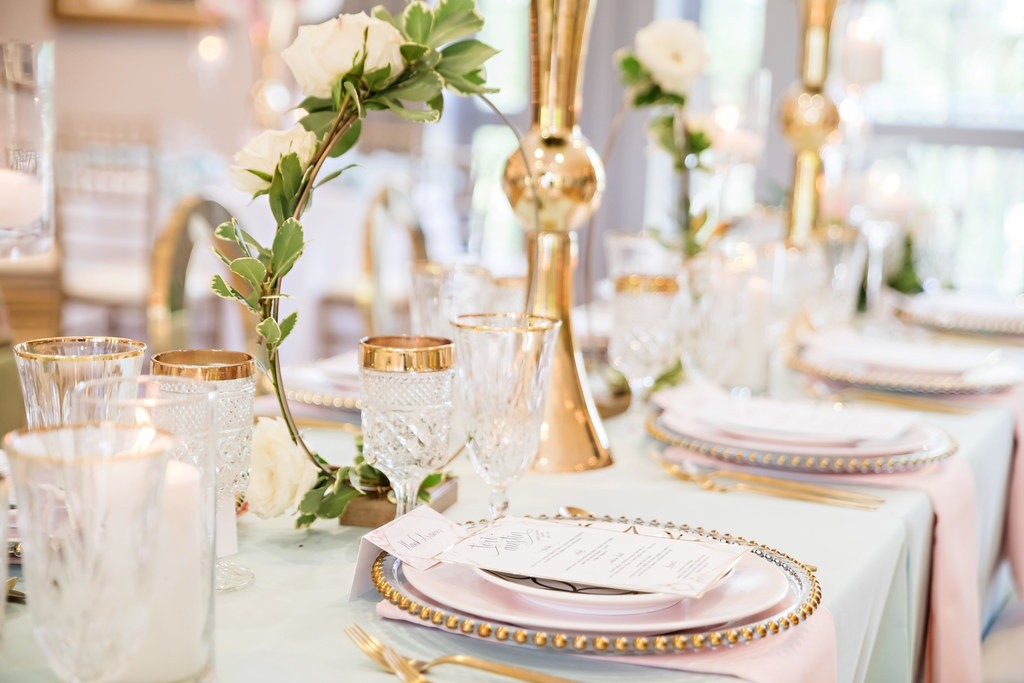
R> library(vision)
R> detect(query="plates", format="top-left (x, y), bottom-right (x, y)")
top-left (281, 369), bottom-right (362, 410)
top-left (703, 413), bottom-right (869, 448)
top-left (372, 518), bottom-right (822, 652)
top-left (402, 524), bottom-right (789, 632)
top-left (469, 563), bottom-right (733, 614)
top-left (647, 401), bottom-right (959, 471)
top-left (798, 352), bottom-right (1024, 391)
top-left (659, 407), bottom-right (930, 455)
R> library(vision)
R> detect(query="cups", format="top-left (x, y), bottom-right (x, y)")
top-left (4, 336), bottom-right (253, 678)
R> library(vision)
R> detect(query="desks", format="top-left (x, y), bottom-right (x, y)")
top-left (1, 294), bottom-right (1024, 683)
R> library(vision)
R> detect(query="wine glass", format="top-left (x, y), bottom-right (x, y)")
top-left (453, 315), bottom-right (562, 518)
top-left (608, 274), bottom-right (682, 450)
top-left (354, 330), bottom-right (453, 523)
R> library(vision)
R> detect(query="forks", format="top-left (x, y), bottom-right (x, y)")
top-left (345, 623), bottom-right (578, 682)
top-left (378, 650), bottom-right (434, 682)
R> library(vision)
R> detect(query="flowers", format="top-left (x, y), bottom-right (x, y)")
top-left (200, 1), bottom-right (540, 529)
top-left (617, 18), bottom-right (742, 301)
